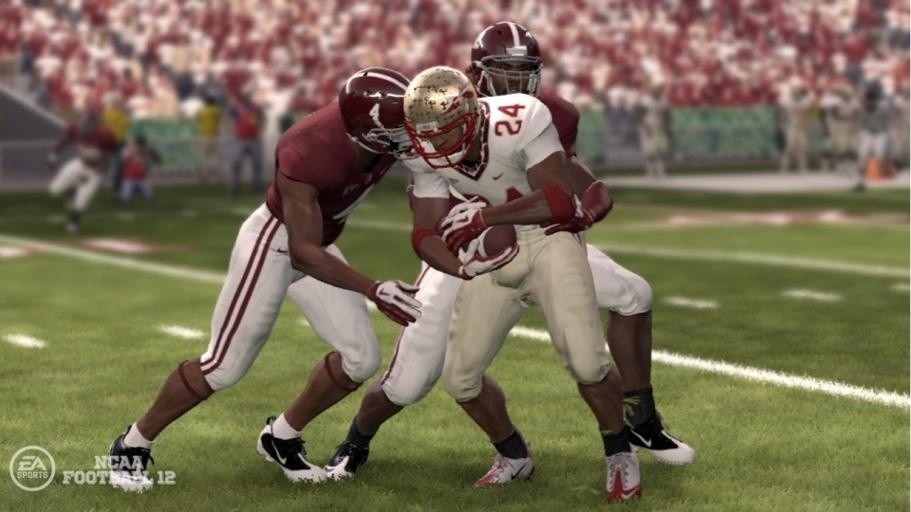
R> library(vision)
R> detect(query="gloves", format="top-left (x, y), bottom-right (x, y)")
top-left (434, 209), bottom-right (488, 257)
top-left (455, 242), bottom-right (521, 281)
top-left (539, 196), bottom-right (597, 235)
top-left (369, 278), bottom-right (422, 328)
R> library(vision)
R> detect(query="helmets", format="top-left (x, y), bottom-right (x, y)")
top-left (338, 67), bottom-right (420, 161)
top-left (467, 22), bottom-right (544, 100)
top-left (403, 66), bottom-right (482, 170)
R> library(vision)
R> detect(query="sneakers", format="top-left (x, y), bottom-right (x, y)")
top-left (473, 454), bottom-right (534, 490)
top-left (322, 440), bottom-right (369, 482)
top-left (604, 443), bottom-right (641, 504)
top-left (256, 417), bottom-right (327, 484)
top-left (628, 420), bottom-right (695, 465)
top-left (107, 426), bottom-right (155, 494)
